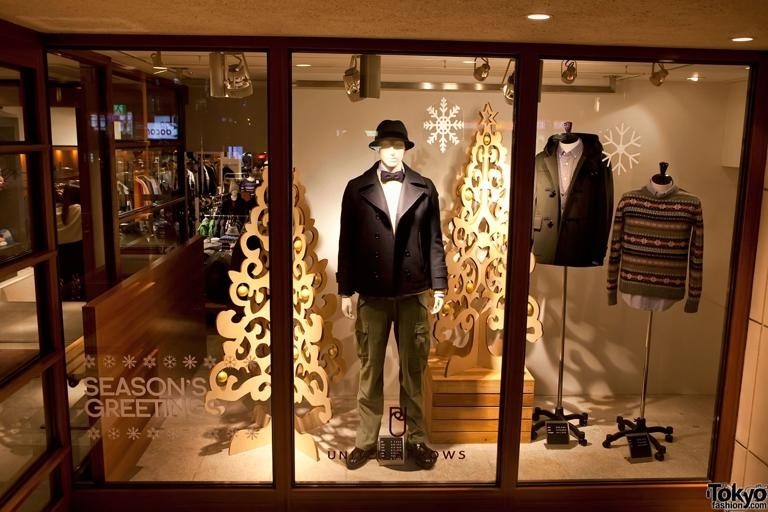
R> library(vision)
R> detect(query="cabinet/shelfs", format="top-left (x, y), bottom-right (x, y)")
top-left (0, 45), bottom-right (768, 512)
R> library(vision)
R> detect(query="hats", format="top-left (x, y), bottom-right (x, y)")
top-left (366, 117), bottom-right (415, 151)
top-left (227, 178), bottom-right (239, 194)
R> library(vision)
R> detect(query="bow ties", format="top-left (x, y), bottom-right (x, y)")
top-left (378, 169), bottom-right (405, 184)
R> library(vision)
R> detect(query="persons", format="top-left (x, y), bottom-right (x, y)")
top-left (217, 179), bottom-right (250, 215)
top-left (334, 119), bottom-right (448, 474)
top-left (531, 128), bottom-right (611, 269)
top-left (54, 185), bottom-right (84, 302)
top-left (602, 173), bottom-right (706, 317)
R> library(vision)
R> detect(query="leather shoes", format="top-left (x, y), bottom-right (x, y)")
top-left (403, 435), bottom-right (438, 471)
top-left (342, 445), bottom-right (379, 472)
